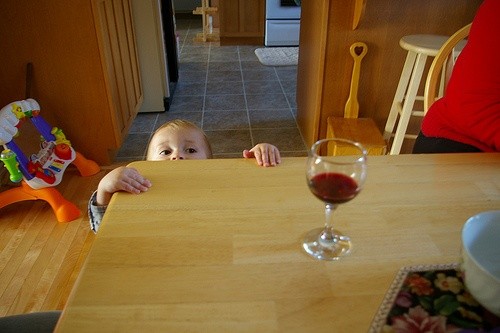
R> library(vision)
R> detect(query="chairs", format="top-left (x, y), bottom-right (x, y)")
top-left (424, 23), bottom-right (473, 117)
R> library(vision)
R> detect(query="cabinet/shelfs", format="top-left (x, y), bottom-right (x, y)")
top-left (192, 0), bottom-right (219, 42)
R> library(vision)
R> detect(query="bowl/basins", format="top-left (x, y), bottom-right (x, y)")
top-left (461, 210), bottom-right (500, 316)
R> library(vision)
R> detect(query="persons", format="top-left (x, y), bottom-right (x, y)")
top-left (409, 0), bottom-right (500, 154)
top-left (86, 119), bottom-right (281, 238)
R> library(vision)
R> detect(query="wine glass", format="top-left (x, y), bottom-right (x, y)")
top-left (299, 137), bottom-right (368, 262)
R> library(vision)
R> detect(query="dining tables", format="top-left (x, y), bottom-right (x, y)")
top-left (51, 152), bottom-right (500, 333)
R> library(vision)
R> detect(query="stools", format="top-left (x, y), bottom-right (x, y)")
top-left (326, 115), bottom-right (386, 155)
top-left (381, 34), bottom-right (470, 155)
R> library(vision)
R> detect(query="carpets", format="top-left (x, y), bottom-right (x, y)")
top-left (254, 47), bottom-right (298, 65)
